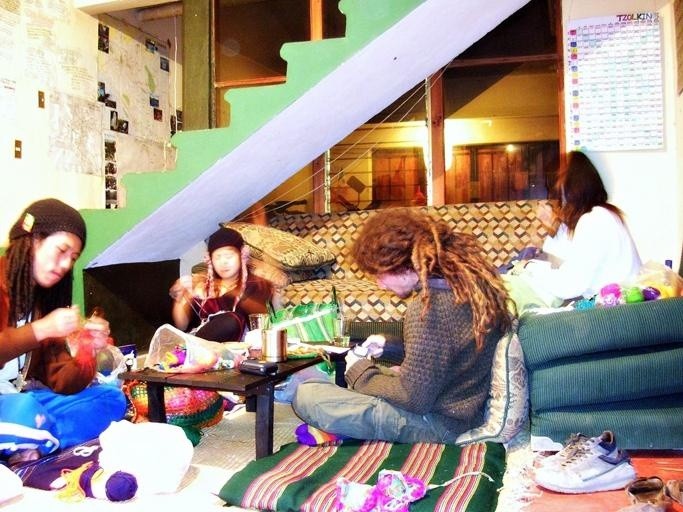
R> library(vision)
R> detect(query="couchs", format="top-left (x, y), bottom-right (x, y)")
top-left (190, 199), bottom-right (559, 338)
top-left (517, 297), bottom-right (683, 454)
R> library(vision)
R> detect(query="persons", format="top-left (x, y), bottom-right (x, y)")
top-left (0, 198), bottom-right (123, 463)
top-left (518, 150), bottom-right (644, 306)
top-left (169, 222), bottom-right (276, 342)
top-left (291, 206), bottom-right (517, 444)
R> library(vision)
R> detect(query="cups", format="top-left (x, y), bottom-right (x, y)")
top-left (248, 312), bottom-right (271, 331)
top-left (261, 328), bottom-right (288, 363)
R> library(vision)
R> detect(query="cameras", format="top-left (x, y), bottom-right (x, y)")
top-left (238, 359), bottom-right (279, 375)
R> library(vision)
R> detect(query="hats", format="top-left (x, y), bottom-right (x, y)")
top-left (10, 199), bottom-right (86, 247)
top-left (209, 227), bottom-right (244, 254)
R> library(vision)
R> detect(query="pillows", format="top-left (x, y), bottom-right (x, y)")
top-left (219, 222), bottom-right (336, 270)
top-left (248, 256), bottom-right (318, 287)
top-left (456, 319), bottom-right (529, 449)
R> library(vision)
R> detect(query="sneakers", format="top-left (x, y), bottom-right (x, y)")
top-left (535, 430), bottom-right (637, 493)
top-left (627, 476), bottom-right (683, 511)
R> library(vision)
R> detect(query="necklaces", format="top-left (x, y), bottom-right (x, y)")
top-left (18, 316), bottom-right (28, 388)
